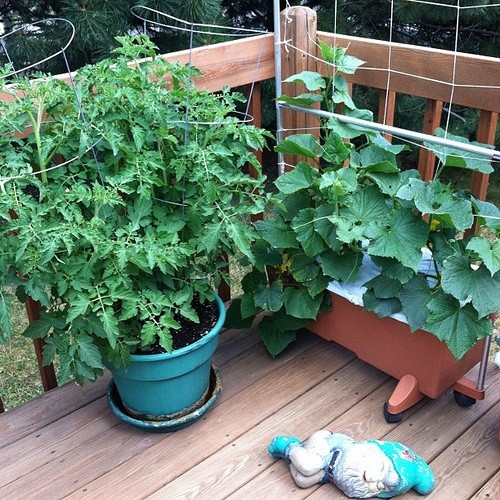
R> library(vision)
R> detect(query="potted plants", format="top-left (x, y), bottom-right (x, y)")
top-left (0, 28), bottom-right (278, 433)
top-left (250, 37), bottom-right (500, 421)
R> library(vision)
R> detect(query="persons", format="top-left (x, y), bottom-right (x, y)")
top-left (268, 428), bottom-right (436, 499)
